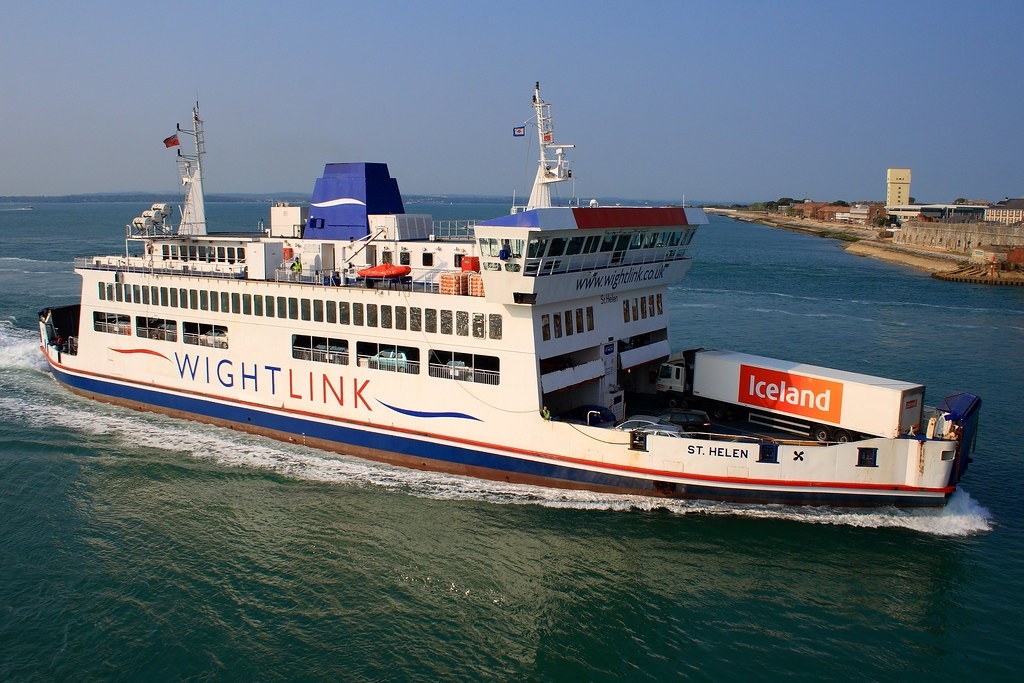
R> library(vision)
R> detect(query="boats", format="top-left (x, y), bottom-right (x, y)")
top-left (36, 79), bottom-right (982, 508)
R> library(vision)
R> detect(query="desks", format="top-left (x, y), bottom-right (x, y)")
top-left (353, 276), bottom-right (413, 290)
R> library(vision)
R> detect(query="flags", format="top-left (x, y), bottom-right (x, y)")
top-left (512, 127), bottom-right (525, 136)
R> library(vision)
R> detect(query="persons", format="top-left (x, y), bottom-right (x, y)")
top-left (290, 257), bottom-right (303, 280)
top-left (504, 239), bottom-right (512, 257)
top-left (163, 134), bottom-right (179, 148)
top-left (540, 406), bottom-right (551, 420)
top-left (58, 335), bottom-right (63, 349)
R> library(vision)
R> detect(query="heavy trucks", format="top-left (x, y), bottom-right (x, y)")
top-left (655, 345), bottom-right (927, 446)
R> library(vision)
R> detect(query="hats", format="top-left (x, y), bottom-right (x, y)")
top-left (295, 257), bottom-right (299, 260)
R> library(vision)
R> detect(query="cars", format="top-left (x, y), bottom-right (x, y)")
top-left (112, 316), bottom-right (131, 332)
top-left (196, 329), bottom-right (228, 349)
top-left (370, 348), bottom-right (409, 373)
top-left (631, 425), bottom-right (690, 438)
top-left (558, 404), bottom-right (617, 429)
top-left (654, 408), bottom-right (712, 440)
top-left (152, 322), bottom-right (186, 342)
top-left (606, 415), bottom-right (673, 431)
